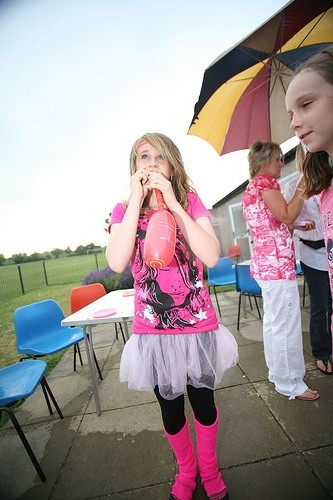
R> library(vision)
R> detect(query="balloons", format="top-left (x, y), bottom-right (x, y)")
top-left (139, 189), bottom-right (177, 269)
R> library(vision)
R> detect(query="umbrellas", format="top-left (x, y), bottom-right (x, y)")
top-left (186, 0), bottom-right (333, 157)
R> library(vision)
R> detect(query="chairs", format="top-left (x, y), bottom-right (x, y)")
top-left (0, 282), bottom-right (125, 484)
top-left (207, 245), bottom-right (305, 329)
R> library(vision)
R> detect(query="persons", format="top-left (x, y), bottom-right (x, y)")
top-left (243, 140), bottom-right (320, 401)
top-left (293, 194), bottom-right (332, 376)
top-left (284, 42), bottom-right (332, 303)
top-left (105, 132), bottom-right (239, 500)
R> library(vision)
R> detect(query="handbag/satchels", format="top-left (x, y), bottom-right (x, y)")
top-left (299, 238), bottom-right (325, 250)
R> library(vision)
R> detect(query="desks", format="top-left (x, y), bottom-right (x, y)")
top-left (61, 289), bottom-right (135, 417)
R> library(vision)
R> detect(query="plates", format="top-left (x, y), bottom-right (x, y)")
top-left (123, 289), bottom-right (135, 295)
top-left (92, 308), bottom-right (115, 317)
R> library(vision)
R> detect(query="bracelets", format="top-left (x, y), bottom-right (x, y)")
top-left (295, 187), bottom-right (306, 193)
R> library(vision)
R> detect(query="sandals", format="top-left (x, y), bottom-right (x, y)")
top-left (297, 389), bottom-right (320, 400)
top-left (316, 356), bottom-right (333, 375)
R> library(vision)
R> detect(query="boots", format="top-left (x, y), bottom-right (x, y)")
top-left (163, 416), bottom-right (198, 500)
top-left (193, 406), bottom-right (229, 500)
